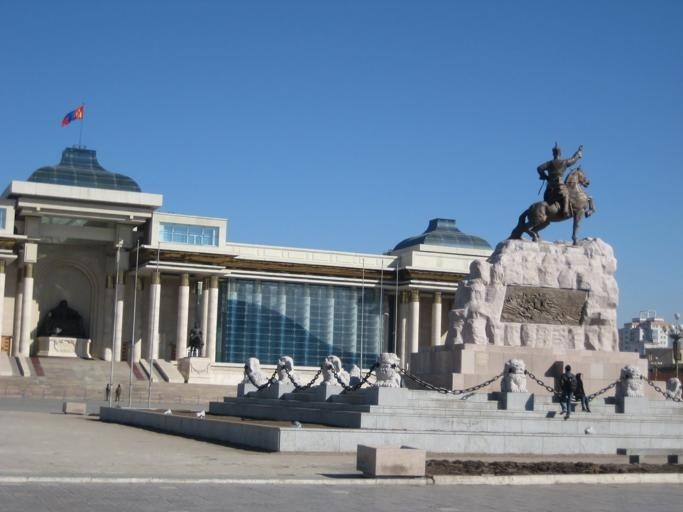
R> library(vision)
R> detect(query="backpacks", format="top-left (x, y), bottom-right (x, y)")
top-left (563, 373), bottom-right (578, 393)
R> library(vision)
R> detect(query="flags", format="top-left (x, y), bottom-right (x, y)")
top-left (61, 106), bottom-right (83, 127)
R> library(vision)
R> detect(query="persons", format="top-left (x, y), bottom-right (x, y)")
top-left (115, 384), bottom-right (121, 401)
top-left (106, 384), bottom-right (111, 401)
top-left (537, 142), bottom-right (584, 216)
top-left (190, 321), bottom-right (204, 346)
top-left (560, 366), bottom-right (591, 419)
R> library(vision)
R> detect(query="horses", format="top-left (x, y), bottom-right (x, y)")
top-left (508, 164), bottom-right (595, 246)
